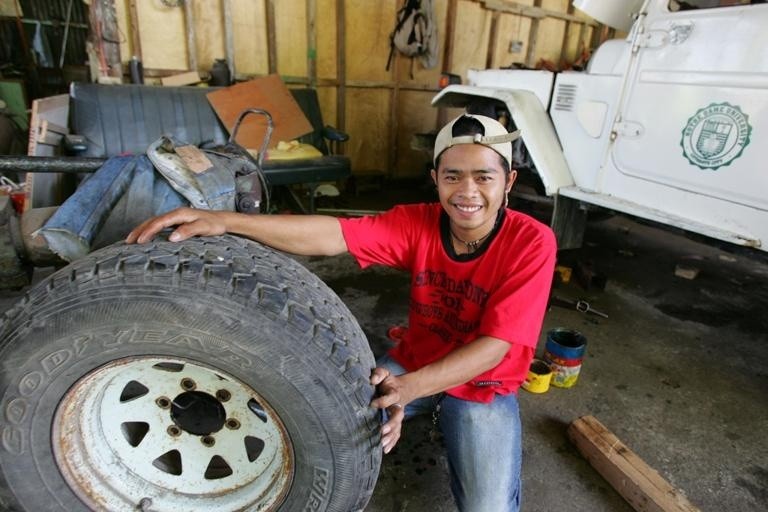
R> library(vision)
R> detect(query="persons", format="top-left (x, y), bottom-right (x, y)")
top-left (125, 113), bottom-right (558, 512)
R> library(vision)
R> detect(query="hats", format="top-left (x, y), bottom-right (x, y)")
top-left (432, 111), bottom-right (522, 210)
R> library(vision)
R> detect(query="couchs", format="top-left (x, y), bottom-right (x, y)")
top-left (64, 82), bottom-right (349, 214)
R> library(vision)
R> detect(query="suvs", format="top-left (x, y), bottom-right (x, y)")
top-left (424, 1), bottom-right (768, 305)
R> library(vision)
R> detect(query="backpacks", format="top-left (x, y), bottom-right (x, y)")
top-left (385, 0), bottom-right (440, 81)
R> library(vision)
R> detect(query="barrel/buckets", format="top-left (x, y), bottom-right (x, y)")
top-left (545, 326), bottom-right (588, 391)
